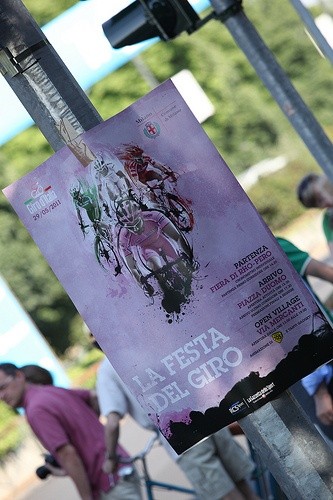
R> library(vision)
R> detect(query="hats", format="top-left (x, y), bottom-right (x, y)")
top-left (82, 322), bottom-right (96, 344)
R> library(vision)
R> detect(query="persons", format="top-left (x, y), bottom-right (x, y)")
top-left (297, 173), bottom-right (333, 257)
top-left (83, 322), bottom-right (264, 500)
top-left (275, 237), bottom-right (333, 322)
top-left (300, 360), bottom-right (333, 428)
top-left (0, 362), bottom-right (143, 500)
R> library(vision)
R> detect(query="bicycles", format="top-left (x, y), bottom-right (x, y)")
top-left (114, 428), bottom-right (274, 500)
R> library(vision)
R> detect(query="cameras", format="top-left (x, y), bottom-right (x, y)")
top-left (36, 455), bottom-right (60, 479)
